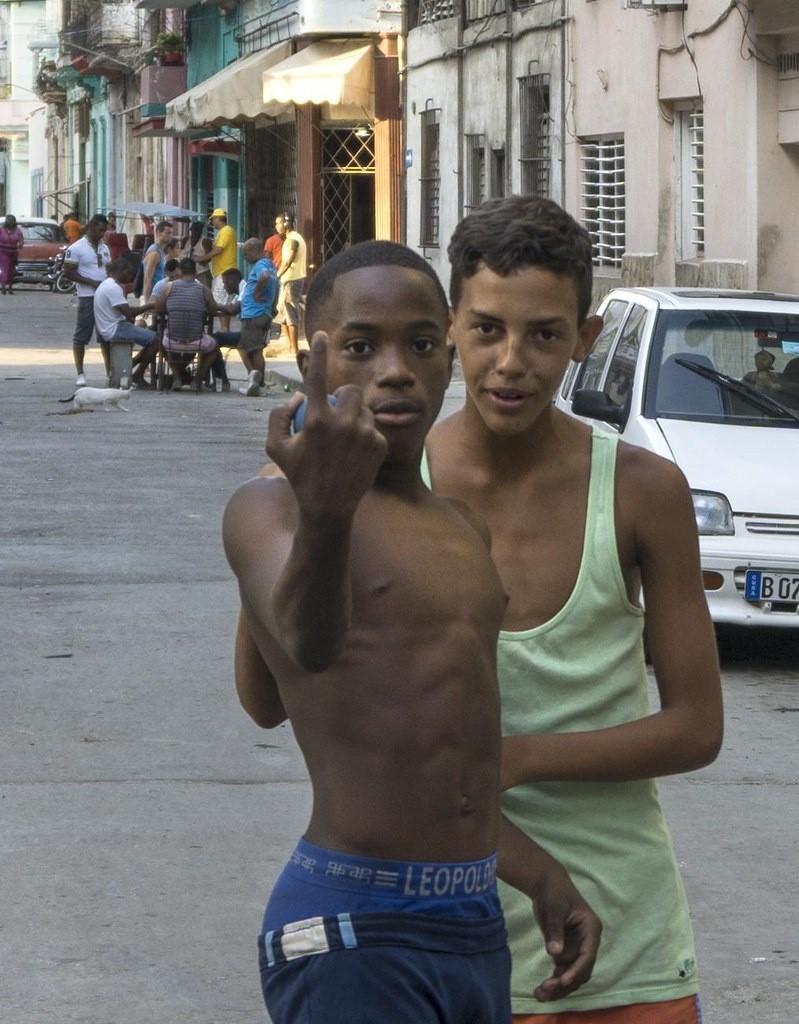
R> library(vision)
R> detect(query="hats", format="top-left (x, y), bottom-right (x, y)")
top-left (209, 208), bottom-right (226, 220)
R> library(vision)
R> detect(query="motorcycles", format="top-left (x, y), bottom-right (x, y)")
top-left (46, 246), bottom-right (77, 293)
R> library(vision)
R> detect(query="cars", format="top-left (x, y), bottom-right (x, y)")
top-left (552, 288), bottom-right (799, 631)
top-left (0, 216), bottom-right (67, 277)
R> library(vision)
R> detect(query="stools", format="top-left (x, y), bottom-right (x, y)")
top-left (108, 339), bottom-right (134, 389)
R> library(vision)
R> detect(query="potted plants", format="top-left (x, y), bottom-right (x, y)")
top-left (156, 32), bottom-right (184, 67)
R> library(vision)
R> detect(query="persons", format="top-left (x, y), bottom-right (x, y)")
top-left (51, 209), bottom-right (307, 397)
top-left (232, 195), bottom-right (724, 1024)
top-left (221, 239), bottom-right (603, 1024)
top-left (0, 215), bottom-right (24, 295)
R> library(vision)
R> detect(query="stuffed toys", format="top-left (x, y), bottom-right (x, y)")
top-left (751, 350), bottom-right (781, 393)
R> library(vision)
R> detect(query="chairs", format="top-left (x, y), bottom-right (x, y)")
top-left (132, 234), bottom-right (155, 252)
top-left (656, 352), bottom-right (725, 416)
top-left (103, 232), bottom-right (129, 261)
top-left (782, 358), bottom-right (799, 407)
top-left (223, 311), bottom-right (278, 385)
top-left (159, 309), bottom-right (210, 396)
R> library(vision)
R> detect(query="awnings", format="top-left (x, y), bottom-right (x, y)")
top-left (165, 39), bottom-right (294, 132)
top-left (264, 39), bottom-right (376, 111)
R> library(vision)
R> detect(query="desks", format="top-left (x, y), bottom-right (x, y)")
top-left (142, 304), bottom-right (237, 392)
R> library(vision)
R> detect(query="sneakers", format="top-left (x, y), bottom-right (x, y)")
top-left (75, 373), bottom-right (85, 385)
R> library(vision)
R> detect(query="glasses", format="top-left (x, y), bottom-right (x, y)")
top-left (97, 253), bottom-right (103, 267)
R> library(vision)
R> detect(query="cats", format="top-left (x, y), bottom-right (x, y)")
top-left (58, 387), bottom-right (134, 412)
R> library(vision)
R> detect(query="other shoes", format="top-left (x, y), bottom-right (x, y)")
top-left (130, 373), bottom-right (150, 388)
top-left (209, 380), bottom-right (230, 392)
top-left (172, 380), bottom-right (182, 391)
top-left (8, 289), bottom-right (13, 294)
top-left (246, 384), bottom-right (258, 397)
top-left (1, 288), bottom-right (6, 294)
top-left (239, 387), bottom-right (248, 395)
top-left (276, 347), bottom-right (299, 357)
top-left (190, 380), bottom-right (201, 391)
